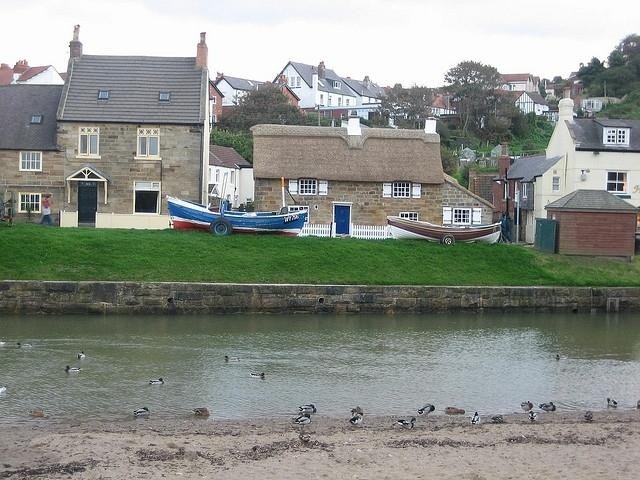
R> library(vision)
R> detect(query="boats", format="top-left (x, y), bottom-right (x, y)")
top-left (166, 194), bottom-right (309, 237)
top-left (387, 215), bottom-right (503, 246)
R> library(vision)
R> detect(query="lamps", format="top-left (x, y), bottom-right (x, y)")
top-left (581, 170), bottom-right (586, 181)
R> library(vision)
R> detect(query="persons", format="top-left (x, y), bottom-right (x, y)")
top-left (499, 211), bottom-right (513, 243)
top-left (38, 196), bottom-right (53, 225)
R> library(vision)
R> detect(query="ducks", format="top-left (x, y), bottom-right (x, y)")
top-left (223, 355), bottom-right (265, 378)
top-left (28, 408), bottom-right (45, 417)
top-left (348, 406), bottom-right (364, 428)
top-left (65, 351), bottom-right (86, 373)
top-left (149, 377), bottom-right (165, 385)
top-left (191, 407), bottom-right (210, 416)
top-left (16, 341), bottom-right (33, 348)
top-left (606, 397), bottom-right (618, 408)
top-left (390, 400), bottom-right (556, 431)
top-left (584, 411), bottom-right (593, 422)
top-left (293, 403), bottom-right (317, 428)
top-left (133, 407), bottom-right (150, 417)
